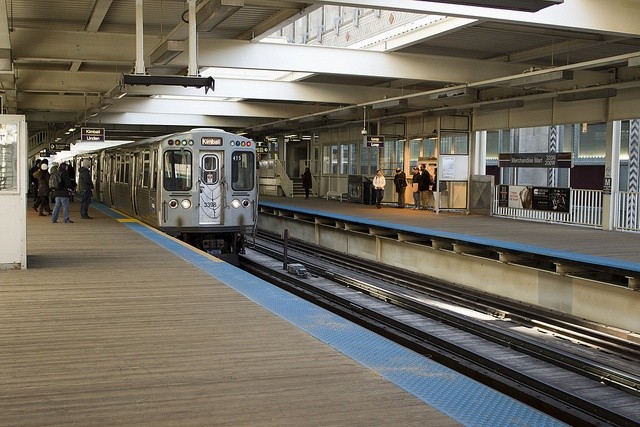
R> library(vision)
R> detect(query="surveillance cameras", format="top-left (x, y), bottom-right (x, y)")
top-left (362, 128), bottom-right (368, 136)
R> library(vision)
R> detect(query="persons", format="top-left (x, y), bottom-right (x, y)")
top-left (32, 159), bottom-right (53, 212)
top-left (49, 163), bottom-right (59, 203)
top-left (302, 167), bottom-right (312, 199)
top-left (30, 159), bottom-right (42, 204)
top-left (38, 164), bottom-right (52, 216)
top-left (52, 163), bottom-right (74, 223)
top-left (432, 168), bottom-right (436, 212)
top-left (78, 159), bottom-right (94, 219)
top-left (67, 165), bottom-right (77, 202)
top-left (394, 168), bottom-right (406, 208)
top-left (418, 164), bottom-right (430, 210)
top-left (411, 167), bottom-right (420, 210)
top-left (373, 170), bottom-right (386, 208)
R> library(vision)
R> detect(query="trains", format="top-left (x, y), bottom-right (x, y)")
top-left (52, 127), bottom-right (259, 234)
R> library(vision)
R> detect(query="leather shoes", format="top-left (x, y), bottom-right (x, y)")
top-left (81, 215), bottom-right (93, 219)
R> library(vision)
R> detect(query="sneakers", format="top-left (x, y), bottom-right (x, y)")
top-left (39, 212), bottom-right (46, 216)
top-left (64, 219), bottom-right (74, 223)
top-left (32, 206), bottom-right (38, 212)
top-left (53, 220), bottom-right (57, 223)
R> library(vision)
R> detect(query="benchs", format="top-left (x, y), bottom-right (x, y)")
top-left (326, 191), bottom-right (349, 203)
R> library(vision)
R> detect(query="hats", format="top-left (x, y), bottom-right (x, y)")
top-left (83, 160), bottom-right (91, 168)
top-left (413, 166), bottom-right (419, 170)
top-left (41, 164), bottom-right (48, 170)
top-left (396, 168), bottom-right (402, 172)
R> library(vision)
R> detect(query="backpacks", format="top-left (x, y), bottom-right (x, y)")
top-left (49, 174), bottom-right (59, 189)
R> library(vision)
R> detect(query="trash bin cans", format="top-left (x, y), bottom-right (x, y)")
top-left (363, 180), bottom-right (376, 204)
